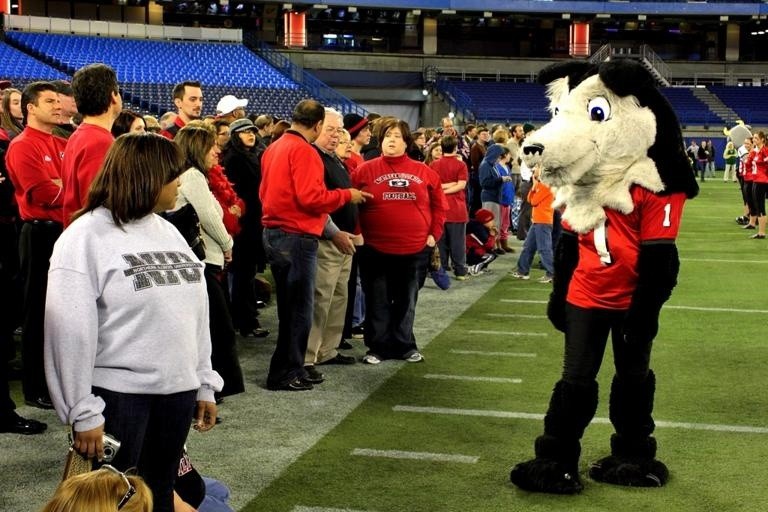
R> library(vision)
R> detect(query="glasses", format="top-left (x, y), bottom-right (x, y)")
top-left (145, 126), bottom-right (161, 134)
top-left (217, 131), bottom-right (228, 137)
top-left (235, 106), bottom-right (248, 113)
top-left (321, 125), bottom-right (346, 135)
top-left (237, 129), bottom-right (257, 135)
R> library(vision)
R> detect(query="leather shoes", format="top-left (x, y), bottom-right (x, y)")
top-left (0, 414), bottom-right (48, 435)
top-left (299, 372), bottom-right (324, 384)
top-left (305, 364), bottom-right (318, 372)
top-left (339, 339), bottom-right (352, 350)
top-left (24, 396), bottom-right (54, 410)
top-left (266, 373), bottom-right (313, 391)
top-left (314, 353), bottom-right (356, 366)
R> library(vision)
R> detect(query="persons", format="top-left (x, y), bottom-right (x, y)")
top-left (343, 112), bottom-right (372, 335)
top-left (2, 87), bottom-right (24, 140)
top-left (428, 136), bottom-right (468, 281)
top-left (511, 163), bottom-right (555, 284)
top-left (112, 109), bottom-right (145, 139)
top-left (62, 62), bottom-right (123, 227)
top-left (208, 137), bottom-right (244, 236)
top-left (1, 97), bottom-right (47, 435)
top-left (255, 114), bottom-right (273, 148)
top-left (479, 144), bottom-right (512, 254)
top-left (158, 126), bottom-right (245, 405)
top-left (698, 140), bottom-right (709, 182)
top-left (165, 82), bottom-right (202, 138)
top-left (723, 142), bottom-right (738, 182)
top-left (490, 123), bottom-right (538, 146)
top-left (5, 81), bottom-right (68, 411)
top-left (748, 131), bottom-right (767, 238)
top-left (215, 93), bottom-right (247, 123)
top-left (274, 120), bottom-right (289, 141)
top-left (304, 106), bottom-right (357, 382)
top-left (707, 139), bottom-right (716, 179)
top-left (686, 139), bottom-right (699, 176)
top-left (221, 118), bottom-right (271, 338)
top-left (143, 116), bottom-right (161, 136)
top-left (736, 138), bottom-right (758, 225)
top-left (336, 125), bottom-right (356, 352)
top-left (43, 132), bottom-right (225, 511)
top-left (469, 130), bottom-right (488, 213)
top-left (44, 466), bottom-right (151, 512)
top-left (174, 443), bottom-right (229, 510)
top-left (355, 118), bottom-right (446, 364)
top-left (259, 99), bottom-right (374, 390)
top-left (410, 119), bottom-right (473, 153)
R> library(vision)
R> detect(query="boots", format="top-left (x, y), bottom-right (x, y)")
top-left (509, 379), bottom-right (602, 495)
top-left (587, 370), bottom-right (670, 489)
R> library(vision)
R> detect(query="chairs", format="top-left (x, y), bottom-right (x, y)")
top-left (658, 86), bottom-right (727, 127)
top-left (0, 30), bottom-right (337, 126)
top-left (429, 79), bottom-right (553, 126)
top-left (705, 83), bottom-right (767, 126)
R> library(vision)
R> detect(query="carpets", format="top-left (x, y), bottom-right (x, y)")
top-left (0, 171), bottom-right (767, 510)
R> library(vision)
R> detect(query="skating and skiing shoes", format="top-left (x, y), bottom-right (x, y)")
top-left (465, 266), bottom-right (483, 277)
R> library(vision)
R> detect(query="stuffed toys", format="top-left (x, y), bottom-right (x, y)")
top-left (509, 59), bottom-right (700, 492)
top-left (725, 120), bottom-right (752, 149)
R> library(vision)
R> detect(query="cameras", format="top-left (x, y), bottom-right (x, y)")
top-left (102, 432), bottom-right (121, 462)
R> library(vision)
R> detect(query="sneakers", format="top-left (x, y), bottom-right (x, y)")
top-left (362, 354), bottom-right (381, 365)
top-left (506, 269), bottom-right (530, 280)
top-left (736, 216), bottom-right (743, 224)
top-left (742, 216), bottom-right (749, 225)
top-left (741, 223), bottom-right (756, 229)
top-left (494, 248), bottom-right (505, 255)
top-left (455, 273), bottom-right (468, 281)
top-left (407, 352), bottom-right (425, 363)
top-left (536, 276), bottom-right (552, 284)
top-left (504, 247), bottom-right (512, 253)
top-left (748, 234), bottom-right (766, 239)
top-left (243, 326), bottom-right (271, 338)
top-left (480, 267), bottom-right (494, 276)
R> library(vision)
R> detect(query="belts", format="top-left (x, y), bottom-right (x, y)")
top-left (24, 218), bottom-right (61, 228)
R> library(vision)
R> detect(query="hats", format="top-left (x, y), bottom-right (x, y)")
top-left (472, 127), bottom-right (489, 140)
top-left (228, 118), bottom-right (259, 137)
top-left (0, 79), bottom-right (11, 90)
top-left (413, 127), bottom-right (426, 135)
top-left (475, 208), bottom-right (495, 225)
top-left (523, 123), bottom-right (536, 134)
top-left (216, 94), bottom-right (249, 117)
top-left (343, 113), bottom-right (368, 139)
